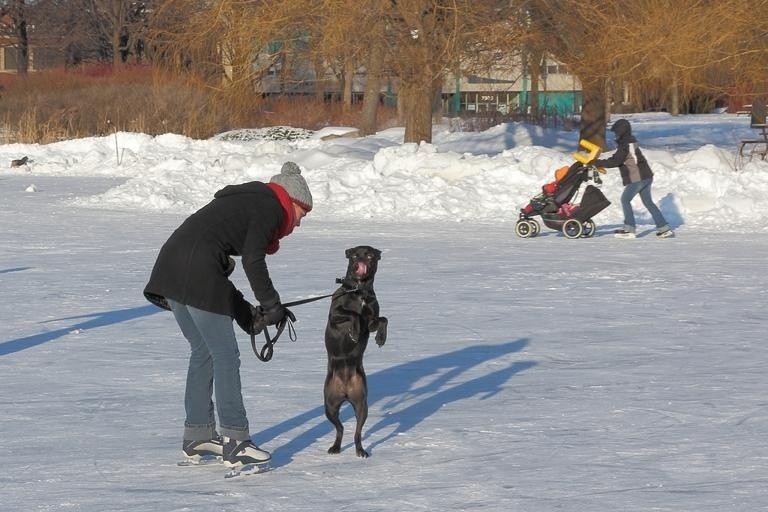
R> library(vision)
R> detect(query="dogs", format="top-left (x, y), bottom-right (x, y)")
top-left (10, 156), bottom-right (29, 168)
top-left (323, 245), bottom-right (388, 459)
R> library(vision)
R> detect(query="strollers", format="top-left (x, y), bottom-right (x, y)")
top-left (512, 155), bottom-right (609, 240)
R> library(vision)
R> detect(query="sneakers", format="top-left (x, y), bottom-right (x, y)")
top-left (220, 434), bottom-right (271, 467)
top-left (182, 432), bottom-right (224, 459)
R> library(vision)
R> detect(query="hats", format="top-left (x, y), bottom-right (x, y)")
top-left (268, 161), bottom-right (313, 212)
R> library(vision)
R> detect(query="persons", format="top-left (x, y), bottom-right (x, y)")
top-left (144, 161), bottom-right (312, 463)
top-left (522, 167), bottom-right (569, 214)
top-left (594, 119), bottom-right (674, 239)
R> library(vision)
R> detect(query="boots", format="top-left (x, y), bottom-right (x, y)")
top-left (612, 227), bottom-right (636, 240)
top-left (655, 228), bottom-right (674, 238)
